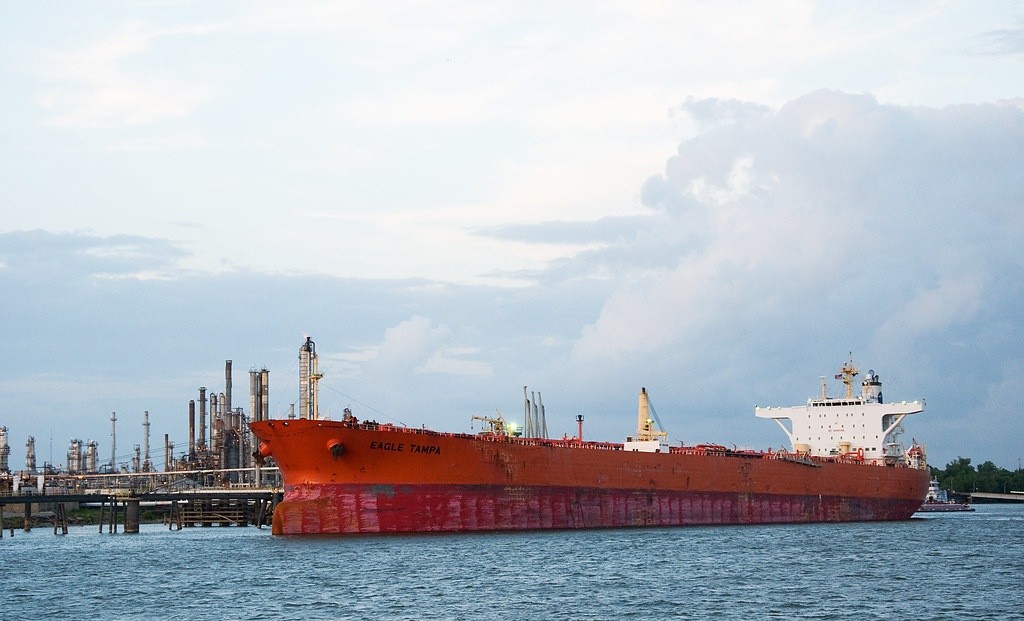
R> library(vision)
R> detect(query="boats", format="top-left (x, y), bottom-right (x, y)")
top-left (907, 438), bottom-right (976, 512)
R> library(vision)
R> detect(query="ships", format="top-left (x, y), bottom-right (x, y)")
top-left (245, 336), bottom-right (933, 537)
